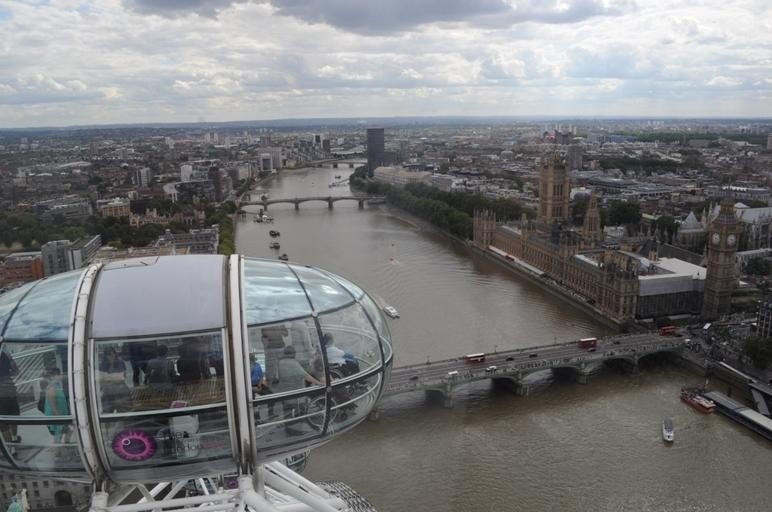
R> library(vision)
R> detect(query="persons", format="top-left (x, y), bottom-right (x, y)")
top-left (38, 368), bottom-right (76, 457)
top-left (98, 334), bottom-right (228, 457)
top-left (249, 325), bottom-right (346, 425)
top-left (1, 346), bottom-right (21, 444)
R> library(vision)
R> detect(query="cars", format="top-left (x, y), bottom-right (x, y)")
top-left (486, 366), bottom-right (496, 372)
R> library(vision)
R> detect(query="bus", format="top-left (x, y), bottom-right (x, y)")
top-left (464, 353), bottom-right (485, 364)
top-left (662, 326), bottom-right (676, 333)
top-left (578, 338), bottom-right (597, 348)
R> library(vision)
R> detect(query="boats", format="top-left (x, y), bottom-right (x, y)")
top-left (278, 253), bottom-right (288, 261)
top-left (271, 241), bottom-right (280, 249)
top-left (681, 388), bottom-right (717, 414)
top-left (662, 419), bottom-right (674, 442)
top-left (384, 305), bottom-right (400, 318)
top-left (269, 230), bottom-right (280, 236)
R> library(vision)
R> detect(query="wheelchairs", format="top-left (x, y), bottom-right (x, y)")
top-left (299, 345), bottom-right (368, 431)
